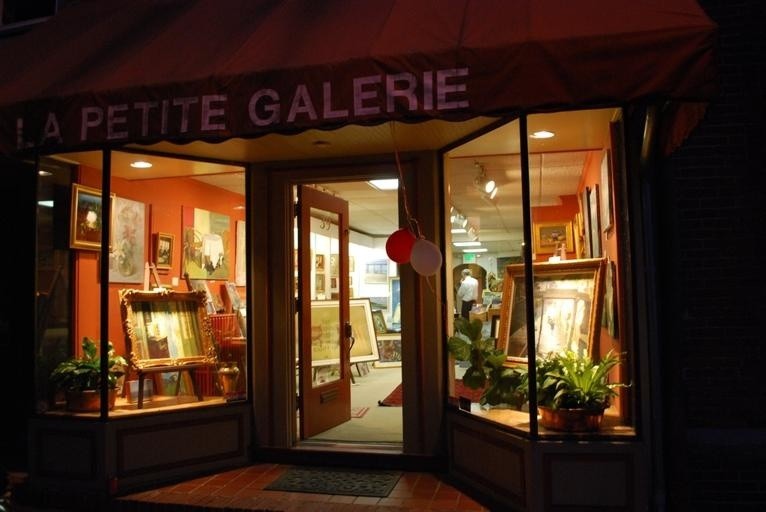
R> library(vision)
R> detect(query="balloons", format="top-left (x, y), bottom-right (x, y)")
top-left (386, 223), bottom-right (417, 266)
top-left (409, 237), bottom-right (442, 276)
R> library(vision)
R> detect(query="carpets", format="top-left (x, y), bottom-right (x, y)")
top-left (296, 376), bottom-right (525, 420)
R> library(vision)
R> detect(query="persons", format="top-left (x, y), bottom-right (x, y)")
top-left (458, 267), bottom-right (479, 318)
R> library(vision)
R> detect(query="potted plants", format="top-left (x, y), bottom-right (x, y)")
top-left (532, 349), bottom-right (634, 435)
top-left (48, 334), bottom-right (130, 416)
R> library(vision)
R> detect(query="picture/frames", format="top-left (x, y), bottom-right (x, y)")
top-left (294, 254), bottom-right (403, 369)
top-left (495, 259), bottom-right (606, 373)
top-left (68, 181), bottom-right (221, 374)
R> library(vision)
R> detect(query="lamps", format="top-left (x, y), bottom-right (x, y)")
top-left (472, 161), bottom-right (500, 201)
